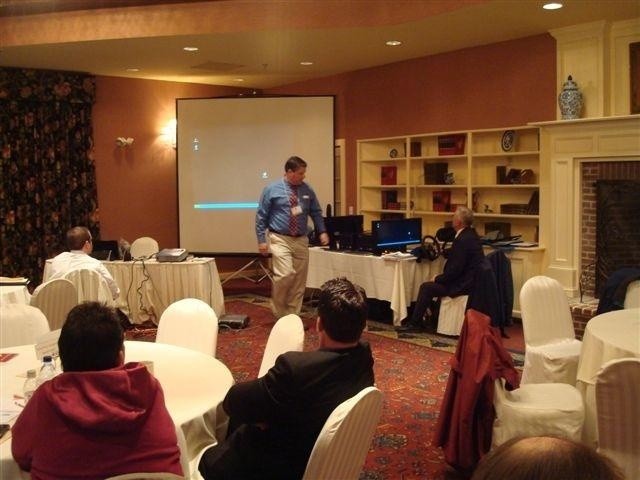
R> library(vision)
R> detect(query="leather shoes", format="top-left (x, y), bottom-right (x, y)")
top-left (395, 323), bottom-right (424, 333)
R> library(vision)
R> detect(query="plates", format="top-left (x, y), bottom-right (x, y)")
top-left (390, 149), bottom-right (398, 157)
top-left (501, 130), bottom-right (516, 152)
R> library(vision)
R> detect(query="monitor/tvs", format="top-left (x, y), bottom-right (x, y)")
top-left (91, 240), bottom-right (119, 260)
top-left (371, 217), bottom-right (422, 253)
top-left (323, 214), bottom-right (363, 252)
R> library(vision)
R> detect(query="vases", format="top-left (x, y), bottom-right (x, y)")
top-left (557, 72), bottom-right (587, 120)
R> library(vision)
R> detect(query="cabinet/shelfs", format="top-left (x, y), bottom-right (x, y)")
top-left (354, 124), bottom-right (543, 245)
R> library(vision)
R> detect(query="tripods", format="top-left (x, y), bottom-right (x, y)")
top-left (219, 257), bottom-right (274, 288)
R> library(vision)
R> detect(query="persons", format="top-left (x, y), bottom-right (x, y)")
top-left (11, 301), bottom-right (186, 480)
top-left (468, 434), bottom-right (627, 480)
top-left (255, 155), bottom-right (330, 332)
top-left (48, 225), bottom-right (121, 301)
top-left (395, 203), bottom-right (486, 334)
top-left (199, 274), bottom-right (376, 479)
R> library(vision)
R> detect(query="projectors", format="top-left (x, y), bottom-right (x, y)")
top-left (156, 247), bottom-right (189, 263)
top-left (219, 314), bottom-right (250, 329)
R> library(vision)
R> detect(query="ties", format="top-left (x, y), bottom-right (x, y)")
top-left (289, 186), bottom-right (298, 237)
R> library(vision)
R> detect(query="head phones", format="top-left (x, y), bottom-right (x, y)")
top-left (420, 235), bottom-right (441, 261)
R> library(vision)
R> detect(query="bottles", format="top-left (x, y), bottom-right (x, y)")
top-left (23, 370), bottom-right (40, 402)
top-left (557, 76), bottom-right (585, 120)
top-left (40, 355), bottom-right (58, 382)
